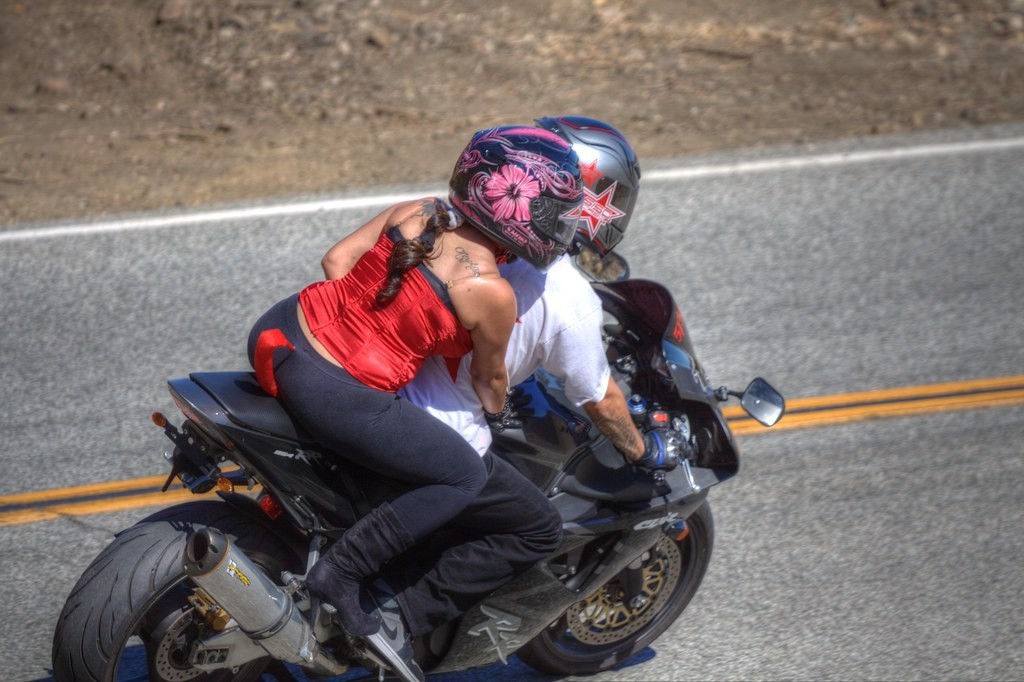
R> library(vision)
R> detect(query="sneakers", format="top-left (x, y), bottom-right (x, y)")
top-left (362, 595), bottom-right (425, 682)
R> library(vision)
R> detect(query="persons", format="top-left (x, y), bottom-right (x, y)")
top-left (360, 113), bottom-right (696, 682)
top-left (245, 125), bottom-right (583, 637)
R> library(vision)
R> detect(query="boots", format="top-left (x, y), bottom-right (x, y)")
top-left (304, 500), bottom-right (415, 637)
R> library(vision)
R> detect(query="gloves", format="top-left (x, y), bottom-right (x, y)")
top-left (636, 427), bottom-right (691, 471)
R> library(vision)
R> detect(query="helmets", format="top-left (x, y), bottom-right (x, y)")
top-left (533, 114), bottom-right (641, 258)
top-left (448, 125), bottom-right (584, 270)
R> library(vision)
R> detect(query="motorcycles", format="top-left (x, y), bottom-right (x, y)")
top-left (51, 243), bottom-right (785, 682)
